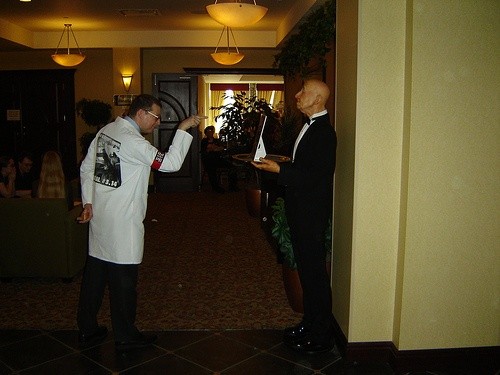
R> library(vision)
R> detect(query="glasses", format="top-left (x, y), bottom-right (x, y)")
top-left (142, 109), bottom-right (161, 121)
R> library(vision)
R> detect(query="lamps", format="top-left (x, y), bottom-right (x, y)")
top-left (210, 23), bottom-right (245, 67)
top-left (121, 74), bottom-right (133, 91)
top-left (50, 23), bottom-right (85, 67)
top-left (205, 0), bottom-right (268, 27)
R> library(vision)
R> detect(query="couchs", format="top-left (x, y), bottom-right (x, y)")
top-left (0, 196), bottom-right (89, 276)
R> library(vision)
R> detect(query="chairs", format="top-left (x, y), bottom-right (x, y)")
top-left (202, 160), bottom-right (226, 193)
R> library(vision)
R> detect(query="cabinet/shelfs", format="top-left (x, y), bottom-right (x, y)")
top-left (0, 68), bottom-right (79, 190)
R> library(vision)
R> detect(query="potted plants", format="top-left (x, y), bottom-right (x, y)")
top-left (208, 84), bottom-right (331, 314)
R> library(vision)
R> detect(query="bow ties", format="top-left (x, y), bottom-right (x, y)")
top-left (303, 116), bottom-right (317, 125)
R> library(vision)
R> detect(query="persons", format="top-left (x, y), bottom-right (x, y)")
top-left (201, 126), bottom-right (227, 193)
top-left (0, 152), bottom-right (71, 200)
top-left (76, 93), bottom-right (208, 351)
top-left (251, 78), bottom-right (339, 356)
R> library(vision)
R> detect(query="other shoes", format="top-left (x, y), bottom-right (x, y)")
top-left (114, 334), bottom-right (158, 351)
top-left (78, 326), bottom-right (109, 347)
top-left (284, 322), bottom-right (334, 353)
